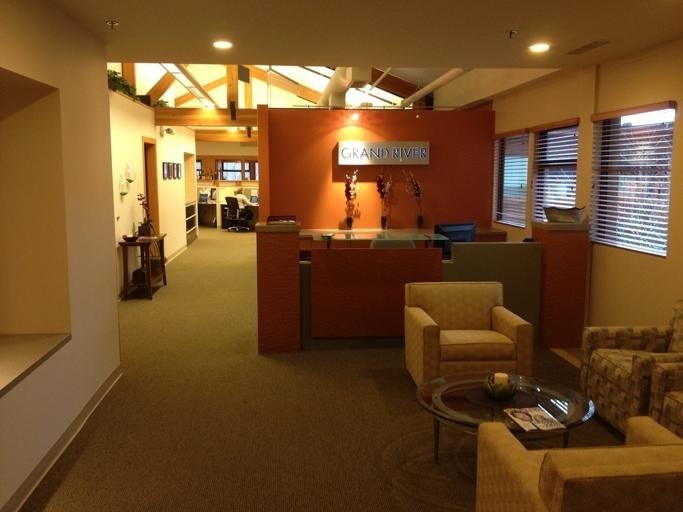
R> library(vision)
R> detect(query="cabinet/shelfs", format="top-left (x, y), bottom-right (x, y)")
top-left (184, 201), bottom-right (197, 247)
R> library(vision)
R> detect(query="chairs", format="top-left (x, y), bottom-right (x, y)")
top-left (223, 196), bottom-right (249, 232)
top-left (578, 300), bottom-right (682, 439)
top-left (402, 281), bottom-right (534, 403)
top-left (474, 416), bottom-right (682, 512)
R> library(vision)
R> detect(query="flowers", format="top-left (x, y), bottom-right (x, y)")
top-left (343, 169), bottom-right (359, 217)
top-left (135, 192), bottom-right (152, 227)
top-left (374, 170), bottom-right (392, 215)
top-left (400, 168), bottom-right (424, 215)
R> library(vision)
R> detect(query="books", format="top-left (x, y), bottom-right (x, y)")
top-left (503, 406), bottom-right (567, 434)
top-left (266, 216), bottom-right (297, 224)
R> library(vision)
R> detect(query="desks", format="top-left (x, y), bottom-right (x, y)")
top-left (220, 201), bottom-right (259, 231)
top-left (117, 233), bottom-right (167, 301)
top-left (198, 199), bottom-right (217, 229)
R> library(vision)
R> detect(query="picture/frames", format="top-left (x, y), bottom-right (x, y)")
top-left (162, 161), bottom-right (181, 180)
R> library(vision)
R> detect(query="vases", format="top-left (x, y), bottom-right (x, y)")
top-left (379, 198), bottom-right (388, 230)
top-left (414, 197), bottom-right (423, 229)
top-left (345, 200), bottom-right (353, 229)
top-left (138, 225), bottom-right (150, 237)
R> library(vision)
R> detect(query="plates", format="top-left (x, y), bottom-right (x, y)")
top-left (122, 235), bottom-right (139, 242)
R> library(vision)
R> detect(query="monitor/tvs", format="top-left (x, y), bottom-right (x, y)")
top-left (201, 194), bottom-right (208, 202)
top-left (250, 196), bottom-right (257, 204)
top-left (434, 222), bottom-right (477, 260)
top-left (210, 189), bottom-right (216, 200)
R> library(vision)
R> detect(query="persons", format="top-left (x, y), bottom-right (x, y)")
top-left (234, 188), bottom-right (258, 232)
